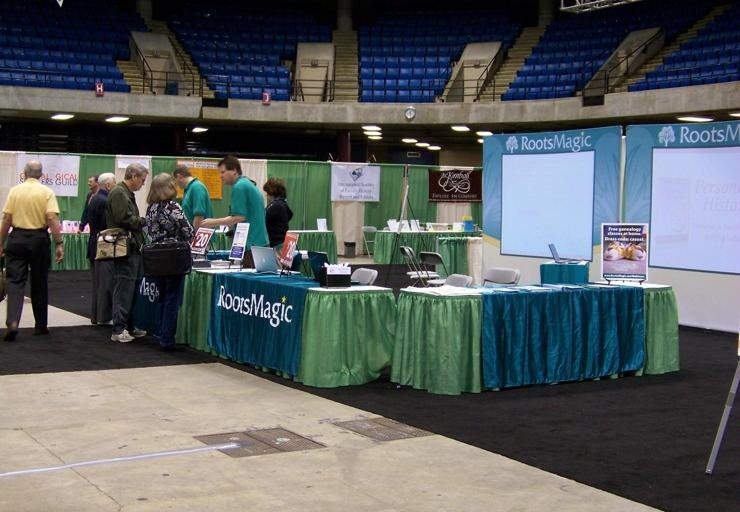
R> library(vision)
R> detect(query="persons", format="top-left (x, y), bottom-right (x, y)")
top-left (87, 172), bottom-right (117, 325)
top-left (173, 166), bottom-right (217, 245)
top-left (145, 174), bottom-right (193, 351)
top-left (0, 160), bottom-right (65, 342)
top-left (263, 177), bottom-right (294, 257)
top-left (103, 163), bottom-right (148, 344)
top-left (198, 155), bottom-right (270, 269)
top-left (76, 175), bottom-right (100, 235)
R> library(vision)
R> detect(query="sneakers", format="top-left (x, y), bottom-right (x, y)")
top-left (92, 319), bottom-right (176, 351)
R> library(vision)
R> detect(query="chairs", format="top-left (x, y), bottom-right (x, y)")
top-left (358, 226), bottom-right (377, 258)
top-left (351, 267), bottom-right (379, 284)
top-left (399, 245), bottom-right (521, 288)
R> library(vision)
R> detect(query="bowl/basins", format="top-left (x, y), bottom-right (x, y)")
top-left (193, 259), bottom-right (211, 267)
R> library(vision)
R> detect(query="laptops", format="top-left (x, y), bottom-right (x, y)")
top-left (549, 244), bottom-right (579, 264)
top-left (251, 245), bottom-right (302, 275)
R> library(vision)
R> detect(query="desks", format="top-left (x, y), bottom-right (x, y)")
top-left (540, 259), bottom-right (590, 284)
top-left (285, 228), bottom-right (481, 278)
top-left (49, 232), bottom-right (93, 273)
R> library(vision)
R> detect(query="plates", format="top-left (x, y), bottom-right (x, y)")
top-left (210, 261), bottom-right (232, 266)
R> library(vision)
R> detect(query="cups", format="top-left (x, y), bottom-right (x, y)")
top-left (387, 220), bottom-right (420, 232)
top-left (317, 219), bottom-right (326, 232)
top-left (427, 216), bottom-right (474, 232)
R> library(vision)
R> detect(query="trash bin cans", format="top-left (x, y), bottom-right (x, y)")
top-left (344, 242), bottom-right (355, 258)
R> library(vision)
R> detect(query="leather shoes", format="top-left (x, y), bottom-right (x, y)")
top-left (3, 327), bottom-right (18, 342)
top-left (35, 328), bottom-right (49, 335)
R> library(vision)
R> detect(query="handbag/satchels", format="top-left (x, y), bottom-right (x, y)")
top-left (142, 242), bottom-right (192, 277)
top-left (95, 229), bottom-right (131, 260)
top-left (0, 254), bottom-right (7, 302)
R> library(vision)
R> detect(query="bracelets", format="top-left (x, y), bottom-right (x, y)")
top-left (56, 240), bottom-right (64, 245)
